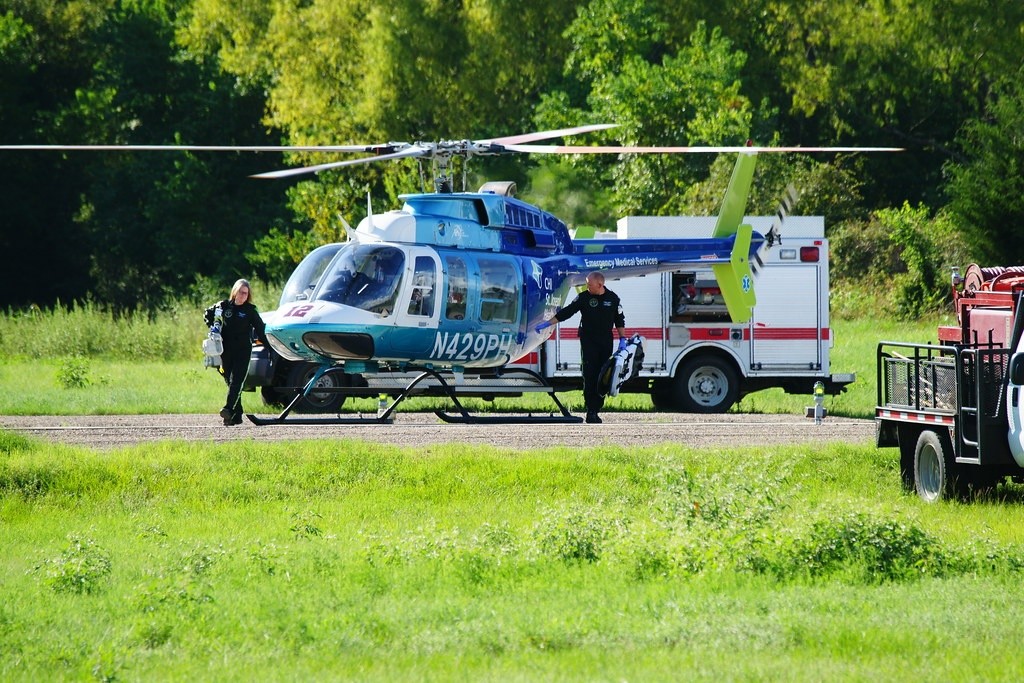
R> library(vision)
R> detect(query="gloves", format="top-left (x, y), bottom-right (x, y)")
top-left (535, 321), bottom-right (552, 335)
top-left (618, 337), bottom-right (627, 350)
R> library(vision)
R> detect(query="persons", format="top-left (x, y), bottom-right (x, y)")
top-left (203, 279), bottom-right (272, 428)
top-left (536, 272), bottom-right (626, 424)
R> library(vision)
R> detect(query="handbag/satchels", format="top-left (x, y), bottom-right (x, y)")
top-left (202, 332), bottom-right (223, 369)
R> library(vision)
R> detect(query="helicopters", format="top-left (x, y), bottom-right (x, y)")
top-left (0, 123), bottom-right (904, 425)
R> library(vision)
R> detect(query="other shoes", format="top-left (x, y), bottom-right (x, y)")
top-left (585, 413), bottom-right (602, 424)
top-left (220, 407), bottom-right (244, 427)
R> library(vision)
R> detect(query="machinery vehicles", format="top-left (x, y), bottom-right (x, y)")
top-left (871, 261), bottom-right (1024, 502)
top-left (203, 215), bottom-right (856, 412)
